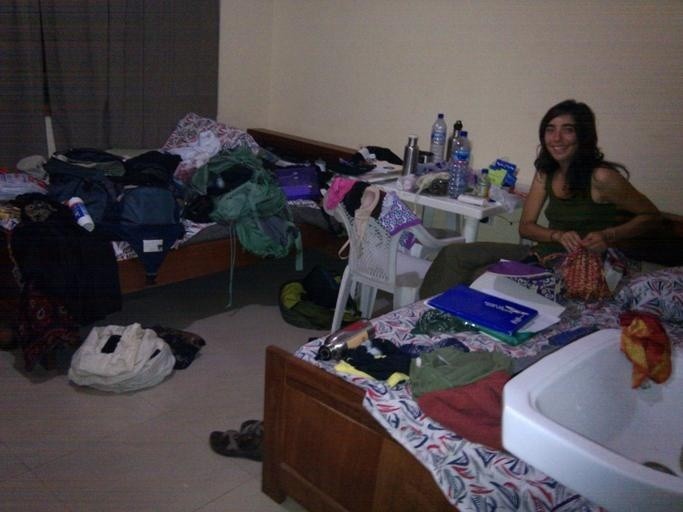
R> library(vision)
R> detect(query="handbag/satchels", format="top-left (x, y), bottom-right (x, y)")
top-left (12, 144), bottom-right (362, 393)
top-left (562, 245), bottom-right (615, 304)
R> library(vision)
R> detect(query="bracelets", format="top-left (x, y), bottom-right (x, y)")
top-left (604, 228), bottom-right (616, 243)
top-left (550, 231), bottom-right (557, 241)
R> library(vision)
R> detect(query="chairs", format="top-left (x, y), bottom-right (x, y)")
top-left (320, 181), bottom-right (467, 342)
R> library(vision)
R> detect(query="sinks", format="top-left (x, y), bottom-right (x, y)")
top-left (502, 326), bottom-right (682, 512)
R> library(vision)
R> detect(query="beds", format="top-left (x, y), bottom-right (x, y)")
top-left (263, 211), bottom-right (683, 512)
top-left (0, 126), bottom-right (360, 373)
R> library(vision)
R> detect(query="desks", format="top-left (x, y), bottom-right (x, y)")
top-left (348, 176), bottom-right (529, 302)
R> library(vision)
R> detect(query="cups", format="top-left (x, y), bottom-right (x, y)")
top-left (418, 149), bottom-right (433, 174)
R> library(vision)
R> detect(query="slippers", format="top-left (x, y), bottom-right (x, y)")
top-left (210, 420), bottom-right (263, 462)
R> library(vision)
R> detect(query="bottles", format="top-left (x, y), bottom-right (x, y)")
top-left (400, 135), bottom-right (419, 177)
top-left (429, 112), bottom-right (446, 165)
top-left (448, 130), bottom-right (471, 199)
top-left (314, 316), bottom-right (375, 363)
top-left (475, 168), bottom-right (491, 198)
top-left (445, 119), bottom-right (464, 161)
top-left (67, 196), bottom-right (96, 232)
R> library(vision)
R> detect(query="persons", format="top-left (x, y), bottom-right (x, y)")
top-left (419, 100), bottom-right (665, 300)
